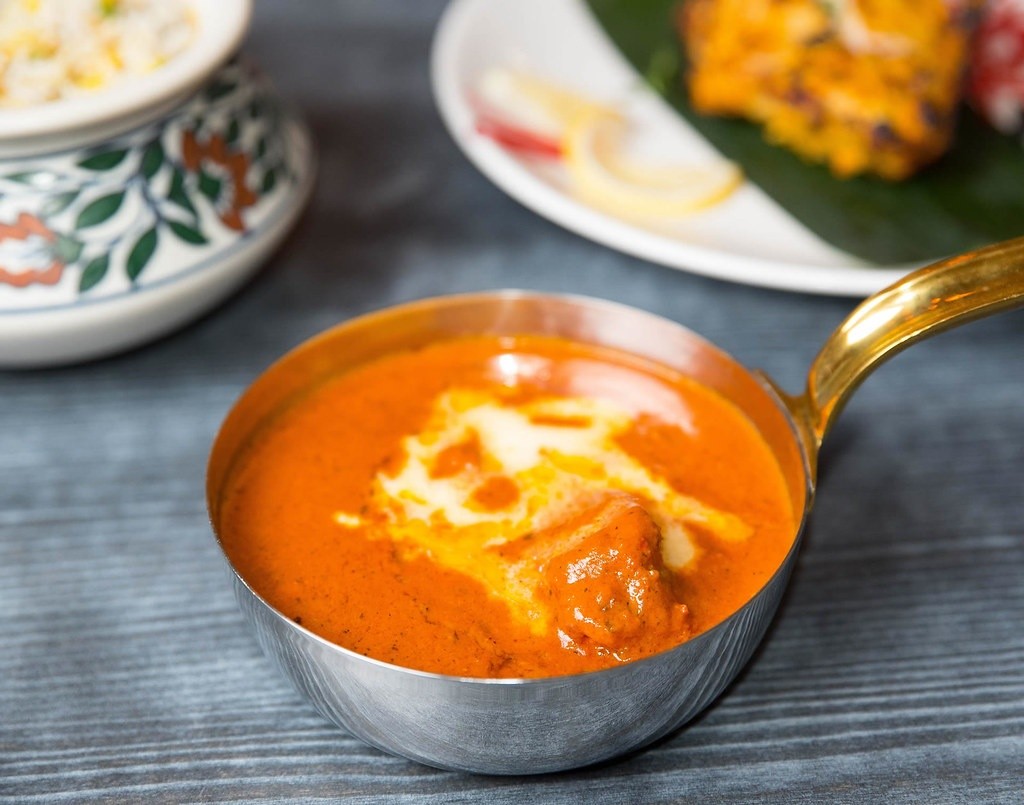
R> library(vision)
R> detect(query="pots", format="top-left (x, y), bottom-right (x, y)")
top-left (208, 239), bottom-right (1024, 778)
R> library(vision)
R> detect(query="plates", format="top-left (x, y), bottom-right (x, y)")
top-left (423, 2), bottom-right (1024, 295)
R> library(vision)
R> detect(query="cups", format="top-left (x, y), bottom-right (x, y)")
top-left (3, 2), bottom-right (312, 375)
